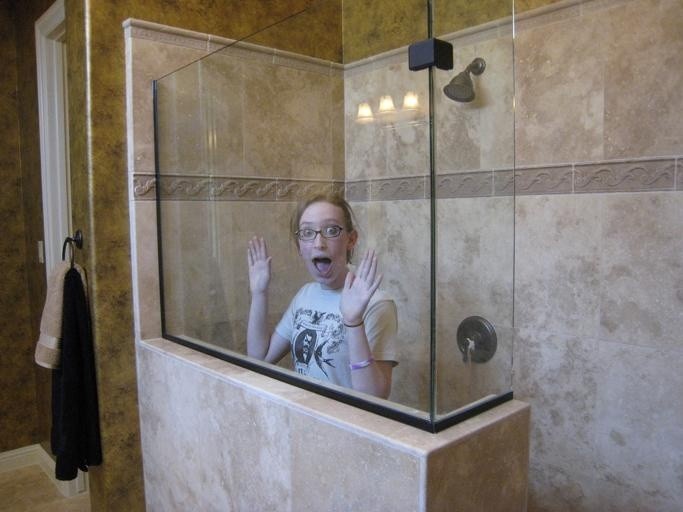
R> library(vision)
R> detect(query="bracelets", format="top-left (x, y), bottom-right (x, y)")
top-left (350, 356), bottom-right (375, 370)
top-left (344, 320), bottom-right (364, 327)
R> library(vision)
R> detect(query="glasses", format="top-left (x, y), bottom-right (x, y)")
top-left (294, 224), bottom-right (344, 243)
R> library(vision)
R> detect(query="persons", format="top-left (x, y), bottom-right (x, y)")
top-left (247, 194), bottom-right (401, 399)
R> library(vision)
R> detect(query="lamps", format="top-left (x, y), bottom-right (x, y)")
top-left (356, 102), bottom-right (373, 122)
top-left (378, 95), bottom-right (396, 114)
top-left (402, 91), bottom-right (420, 111)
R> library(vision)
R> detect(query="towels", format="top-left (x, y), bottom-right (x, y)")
top-left (34, 261), bottom-right (88, 370)
